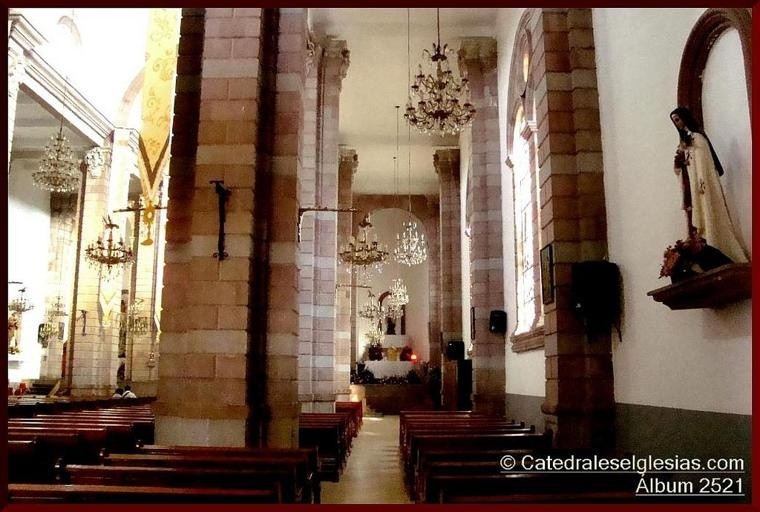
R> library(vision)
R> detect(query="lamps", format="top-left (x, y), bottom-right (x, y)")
top-left (337, 8), bottom-right (476, 346)
top-left (8, 76), bottom-right (156, 339)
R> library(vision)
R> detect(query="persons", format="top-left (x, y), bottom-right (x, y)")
top-left (14, 383), bottom-right (31, 396)
top-left (669, 104), bottom-right (750, 263)
top-left (425, 367), bottom-right (441, 409)
top-left (111, 387), bottom-right (123, 398)
top-left (63, 383), bottom-right (72, 396)
top-left (56, 383), bottom-right (63, 397)
top-left (121, 384), bottom-right (137, 399)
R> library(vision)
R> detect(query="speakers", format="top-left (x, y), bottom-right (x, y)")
top-left (568, 260), bottom-right (624, 334)
top-left (489, 310), bottom-right (507, 336)
top-left (448, 340), bottom-right (464, 361)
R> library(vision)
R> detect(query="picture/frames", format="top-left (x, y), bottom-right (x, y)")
top-left (539, 243), bottom-right (555, 306)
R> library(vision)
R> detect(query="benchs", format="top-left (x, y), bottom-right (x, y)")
top-left (8, 395), bottom-right (751, 504)
